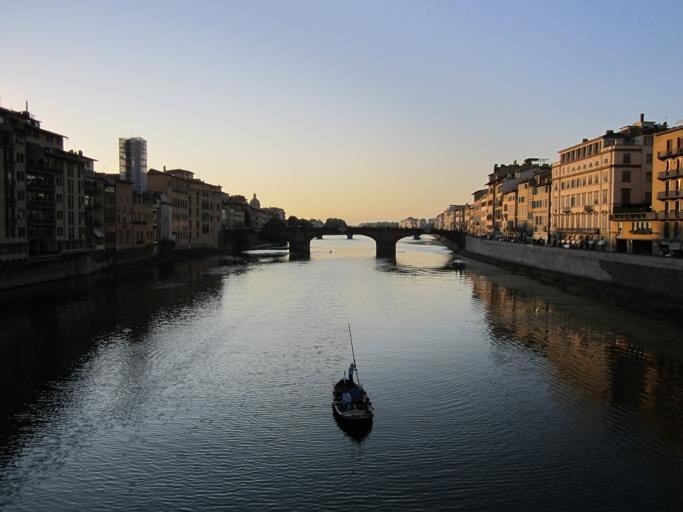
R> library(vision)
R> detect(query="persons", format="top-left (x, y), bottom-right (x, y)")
top-left (342, 390), bottom-right (353, 410)
top-left (349, 363), bottom-right (357, 382)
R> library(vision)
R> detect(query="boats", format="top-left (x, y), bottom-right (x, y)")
top-left (333, 377), bottom-right (375, 424)
